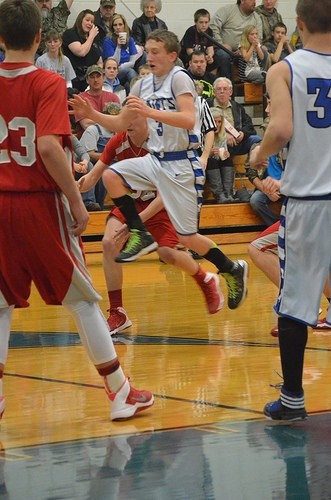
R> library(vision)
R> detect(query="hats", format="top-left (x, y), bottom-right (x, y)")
top-left (87, 64), bottom-right (102, 76)
top-left (100, 0), bottom-right (115, 7)
top-left (103, 102), bottom-right (123, 115)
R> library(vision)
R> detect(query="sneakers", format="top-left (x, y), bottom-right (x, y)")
top-left (107, 307), bottom-right (132, 335)
top-left (217, 259), bottom-right (249, 309)
top-left (103, 375), bottom-right (154, 421)
top-left (312, 317), bottom-right (331, 329)
top-left (114, 227), bottom-right (158, 263)
top-left (196, 272), bottom-right (224, 314)
top-left (264, 389), bottom-right (308, 422)
top-left (271, 306), bottom-right (322, 336)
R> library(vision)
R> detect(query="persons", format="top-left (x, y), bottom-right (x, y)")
top-left (131, 0), bottom-right (167, 48)
top-left (76, 97), bottom-right (224, 335)
top-left (70, 134), bottom-right (100, 212)
top-left (247, 0), bottom-right (331, 421)
top-left (61, 9), bottom-right (104, 92)
top-left (159, 98), bottom-right (216, 264)
top-left (99, 13), bottom-right (150, 81)
top-left (255, 0), bottom-right (283, 41)
top-left (210, 0), bottom-right (262, 53)
top-left (95, 0), bottom-right (116, 30)
top-left (249, 91), bottom-right (331, 337)
top-left (85, 58), bottom-right (126, 104)
top-left (34, 29), bottom-right (76, 88)
top-left (179, 9), bottom-right (304, 228)
top-left (69, 30), bottom-right (249, 308)
top-left (130, 77), bottom-right (142, 89)
top-left (34, 0), bottom-right (74, 57)
top-left (137, 63), bottom-right (152, 76)
top-left (80, 101), bottom-right (123, 210)
top-left (0, 0), bottom-right (154, 421)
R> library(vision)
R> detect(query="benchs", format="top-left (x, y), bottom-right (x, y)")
top-left (76, 59), bottom-right (273, 240)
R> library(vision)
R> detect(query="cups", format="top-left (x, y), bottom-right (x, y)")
top-left (218, 147), bottom-right (227, 161)
top-left (118, 32), bottom-right (127, 44)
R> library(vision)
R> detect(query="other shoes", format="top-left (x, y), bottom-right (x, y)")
top-left (86, 202), bottom-right (102, 211)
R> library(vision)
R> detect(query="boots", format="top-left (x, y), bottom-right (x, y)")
top-left (220, 167), bottom-right (240, 202)
top-left (206, 168), bottom-right (231, 203)
